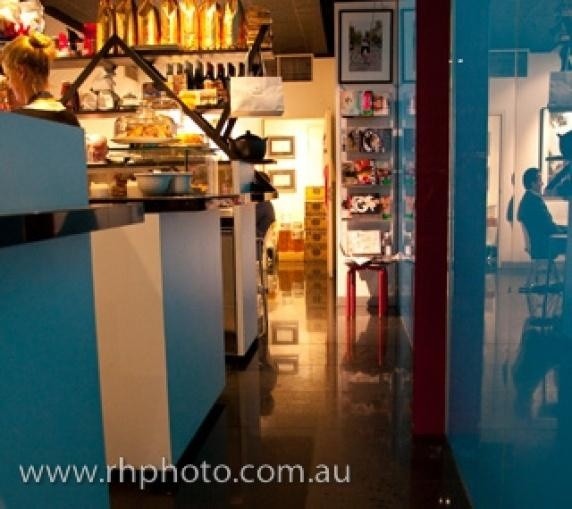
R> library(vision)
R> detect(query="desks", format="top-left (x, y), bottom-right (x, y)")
top-left (95, 191), bottom-right (241, 215)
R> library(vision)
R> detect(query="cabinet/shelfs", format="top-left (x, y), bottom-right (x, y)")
top-left (342, 109), bottom-right (390, 223)
top-left (50, 47), bottom-right (279, 178)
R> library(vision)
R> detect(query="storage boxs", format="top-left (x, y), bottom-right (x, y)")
top-left (302, 180), bottom-right (329, 268)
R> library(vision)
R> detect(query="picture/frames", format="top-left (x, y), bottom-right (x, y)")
top-left (336, 5), bottom-right (395, 87)
top-left (400, 5), bottom-right (415, 87)
top-left (266, 133), bottom-right (297, 162)
top-left (269, 167), bottom-right (300, 195)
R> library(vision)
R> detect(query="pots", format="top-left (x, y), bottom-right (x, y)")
top-left (229, 130), bottom-right (269, 161)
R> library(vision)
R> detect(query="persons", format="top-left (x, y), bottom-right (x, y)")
top-left (518, 167), bottom-right (568, 260)
top-left (507, 327), bottom-right (563, 417)
top-left (259, 351), bottom-right (282, 417)
top-left (505, 169), bottom-right (514, 223)
top-left (256, 171), bottom-right (275, 261)
top-left (351, 31), bottom-right (381, 67)
top-left (0, 32), bottom-right (80, 128)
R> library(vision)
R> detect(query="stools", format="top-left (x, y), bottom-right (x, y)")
top-left (344, 258), bottom-right (389, 320)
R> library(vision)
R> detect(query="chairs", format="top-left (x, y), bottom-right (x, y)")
top-left (515, 219), bottom-right (562, 294)
top-left (257, 222), bottom-right (277, 343)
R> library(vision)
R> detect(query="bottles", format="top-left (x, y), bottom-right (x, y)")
top-left (165, 61), bottom-right (246, 99)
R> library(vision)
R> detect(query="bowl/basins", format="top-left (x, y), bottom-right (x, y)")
top-left (134, 172), bottom-right (192, 193)
